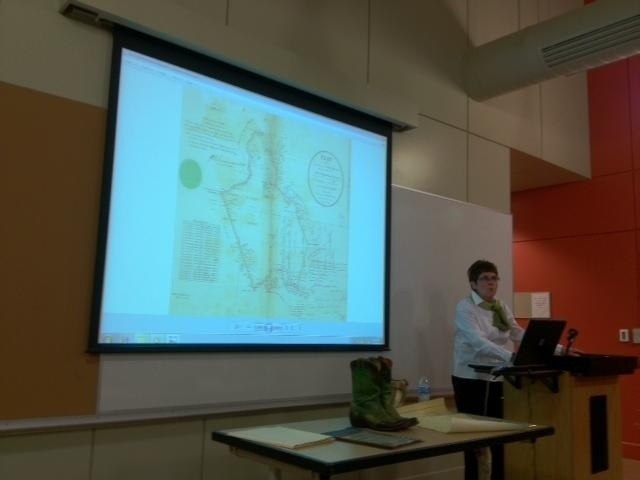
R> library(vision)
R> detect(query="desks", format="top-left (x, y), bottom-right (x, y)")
top-left (210, 411), bottom-right (556, 480)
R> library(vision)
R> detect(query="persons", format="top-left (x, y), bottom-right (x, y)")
top-left (451, 261), bottom-right (583, 480)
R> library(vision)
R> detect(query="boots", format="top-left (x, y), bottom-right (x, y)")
top-left (349, 356), bottom-right (418, 431)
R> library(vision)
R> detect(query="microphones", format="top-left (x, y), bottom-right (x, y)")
top-left (568, 328), bottom-right (578, 342)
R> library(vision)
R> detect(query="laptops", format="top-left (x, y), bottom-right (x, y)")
top-left (468, 319), bottom-right (566, 371)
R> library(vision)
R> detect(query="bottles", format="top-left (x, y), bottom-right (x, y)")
top-left (416, 376), bottom-right (431, 404)
